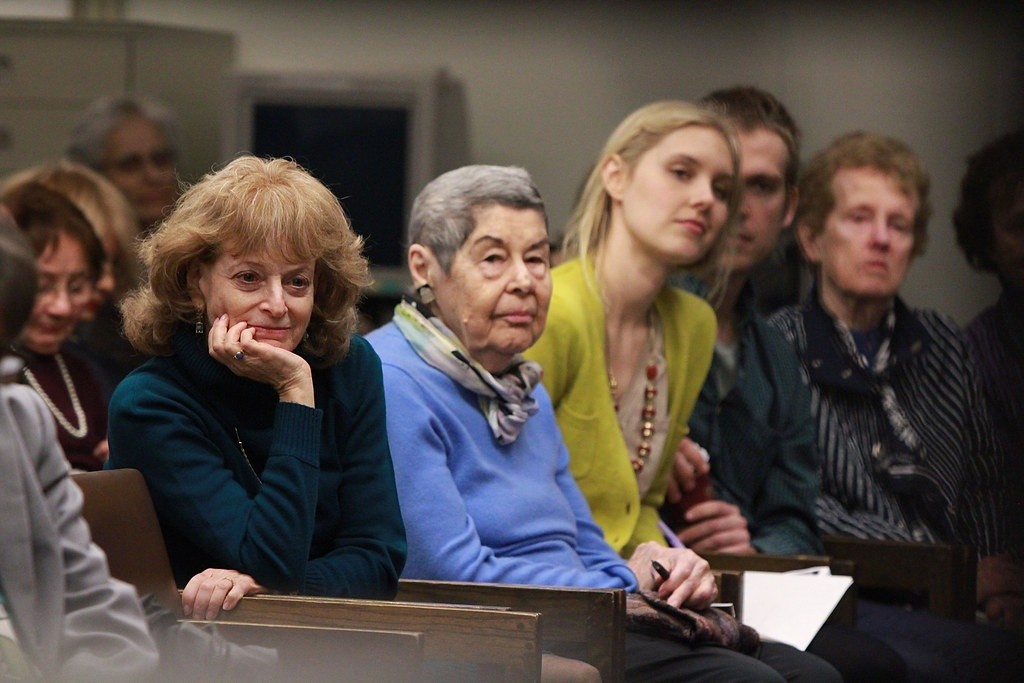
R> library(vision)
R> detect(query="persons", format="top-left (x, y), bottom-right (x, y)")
top-left (0, 216), bottom-right (162, 683)
top-left (520, 100), bottom-right (741, 562)
top-left (952, 130), bottom-right (1024, 464)
top-left (0, 92), bottom-right (179, 471)
top-left (362, 165), bottom-right (718, 611)
top-left (721, 133), bottom-right (1024, 620)
top-left (669, 87), bottom-right (818, 559)
top-left (106, 155), bottom-right (407, 621)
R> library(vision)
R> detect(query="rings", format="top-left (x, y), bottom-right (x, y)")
top-left (232, 350), bottom-right (244, 360)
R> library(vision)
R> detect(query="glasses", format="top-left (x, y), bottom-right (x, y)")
top-left (37, 279), bottom-right (95, 305)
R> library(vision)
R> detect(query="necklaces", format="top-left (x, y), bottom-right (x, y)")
top-left (606, 357), bottom-right (658, 476)
top-left (235, 427), bottom-right (262, 484)
top-left (10, 345), bottom-right (88, 438)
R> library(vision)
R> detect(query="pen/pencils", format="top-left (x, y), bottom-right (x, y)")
top-left (650, 559), bottom-right (671, 580)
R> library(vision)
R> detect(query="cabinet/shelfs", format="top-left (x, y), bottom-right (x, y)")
top-left (0, 17), bottom-right (233, 193)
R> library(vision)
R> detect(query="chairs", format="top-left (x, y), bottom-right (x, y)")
top-left (70, 467), bottom-right (979, 683)
